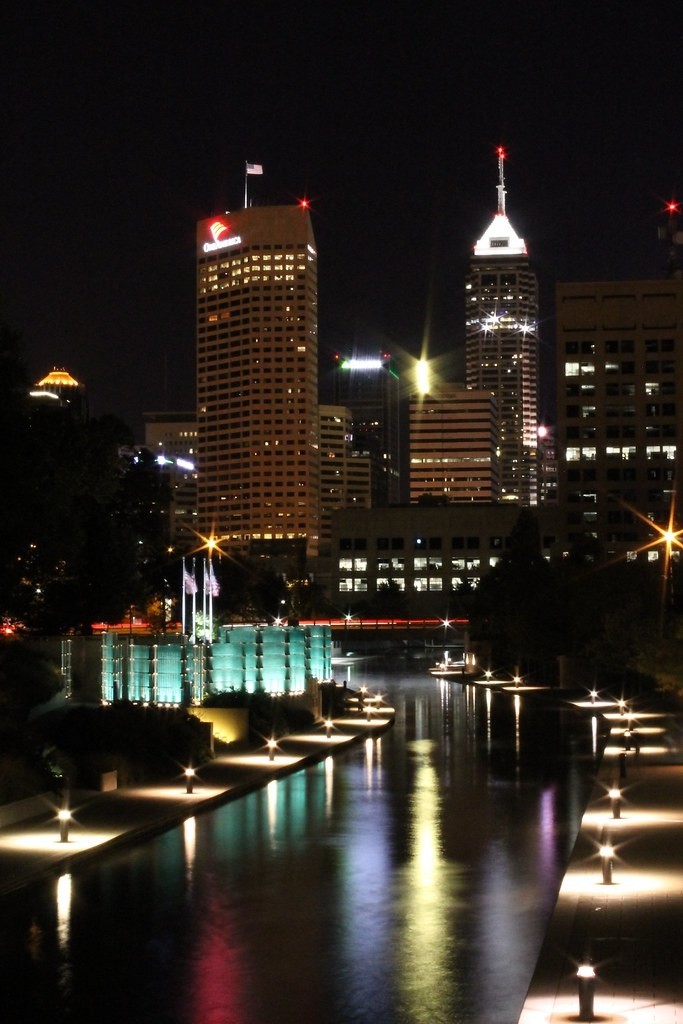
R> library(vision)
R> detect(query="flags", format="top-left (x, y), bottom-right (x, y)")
top-left (246, 163), bottom-right (263, 175)
top-left (184, 570), bottom-right (198, 594)
top-left (204, 565), bottom-right (219, 598)
